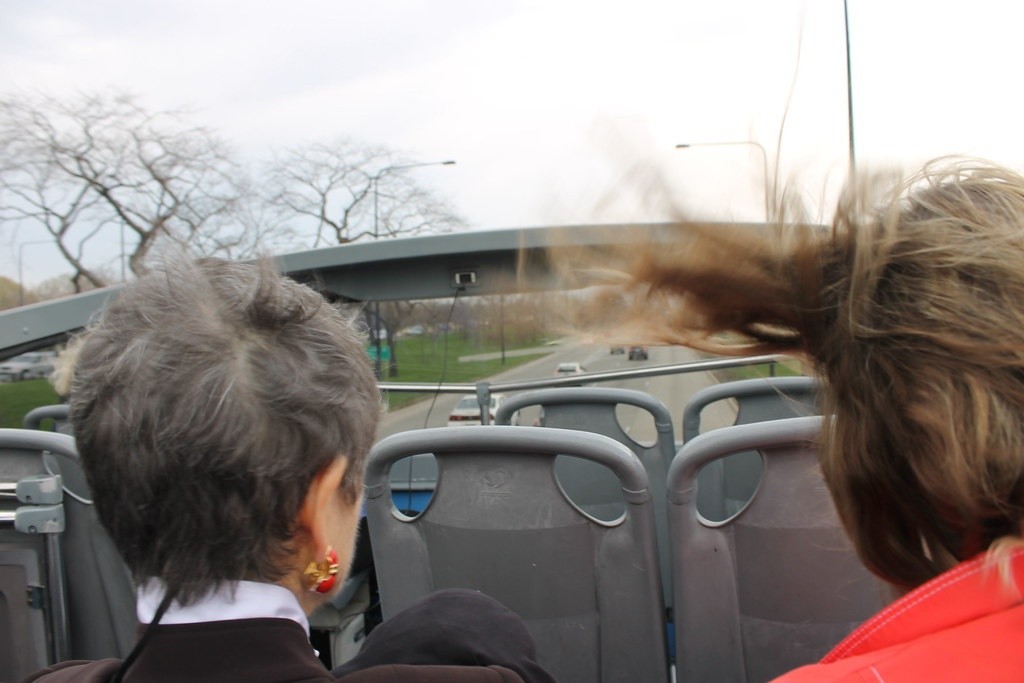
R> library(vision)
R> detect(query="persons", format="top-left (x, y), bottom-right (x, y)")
top-left (645, 170), bottom-right (1024, 683)
top-left (18, 257), bottom-right (557, 683)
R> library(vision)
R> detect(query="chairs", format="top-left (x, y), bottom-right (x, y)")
top-left (682, 375), bottom-right (827, 523)
top-left (364, 424), bottom-right (669, 682)
top-left (665, 413), bottom-right (893, 683)
top-left (494, 386), bottom-right (677, 664)
top-left (0, 427), bottom-right (146, 683)
top-left (22, 405), bottom-right (77, 436)
